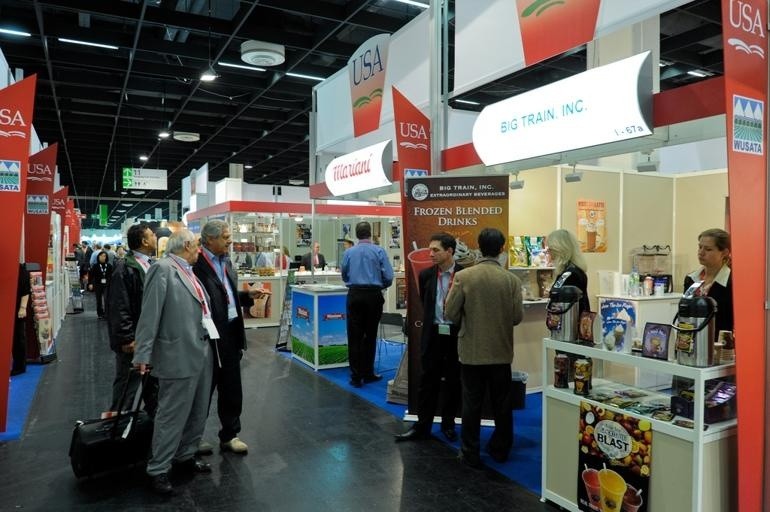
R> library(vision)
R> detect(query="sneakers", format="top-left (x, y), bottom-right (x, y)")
top-left (198, 439), bottom-right (214, 453)
top-left (220, 436), bottom-right (249, 453)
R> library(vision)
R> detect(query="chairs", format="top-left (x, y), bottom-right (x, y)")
top-left (376, 313), bottom-right (406, 375)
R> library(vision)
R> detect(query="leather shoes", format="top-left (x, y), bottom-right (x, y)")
top-left (173, 460), bottom-right (212, 476)
top-left (151, 476), bottom-right (180, 498)
top-left (392, 426), bottom-right (432, 440)
top-left (349, 380), bottom-right (363, 388)
top-left (440, 427), bottom-right (458, 443)
top-left (363, 374), bottom-right (383, 384)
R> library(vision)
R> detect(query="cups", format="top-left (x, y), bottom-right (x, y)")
top-left (714, 330), bottom-right (734, 366)
top-left (582, 468), bottom-right (600, 509)
top-left (621, 483), bottom-right (643, 512)
top-left (597, 468), bottom-right (627, 512)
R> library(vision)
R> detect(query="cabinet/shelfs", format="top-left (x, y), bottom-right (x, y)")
top-left (508, 266), bottom-right (556, 306)
top-left (598, 293), bottom-right (683, 390)
top-left (539, 336), bottom-right (737, 511)
top-left (290, 283), bottom-right (349, 372)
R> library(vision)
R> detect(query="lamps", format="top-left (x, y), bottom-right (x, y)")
top-left (241, 39), bottom-right (286, 68)
top-left (139, 122), bottom-right (150, 163)
top-left (173, 132), bottom-right (200, 144)
top-left (131, 191), bottom-right (146, 195)
top-left (157, 86), bottom-right (172, 138)
top-left (200, 2), bottom-right (220, 82)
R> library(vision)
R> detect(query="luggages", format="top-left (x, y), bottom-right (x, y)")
top-left (67, 364), bottom-right (156, 486)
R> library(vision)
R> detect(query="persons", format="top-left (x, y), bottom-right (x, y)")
top-left (546, 229), bottom-right (590, 313)
top-left (283, 246), bottom-right (293, 269)
top-left (343, 224), bottom-right (351, 240)
top-left (105, 218), bottom-right (272, 496)
top-left (300, 240), bottom-right (325, 271)
top-left (394, 234), bottom-right (463, 439)
top-left (275, 246), bottom-right (286, 270)
top-left (8, 264), bottom-right (43, 379)
top-left (254, 250), bottom-right (268, 267)
top-left (301, 228), bottom-right (311, 239)
top-left (336, 234), bottom-right (354, 272)
top-left (236, 243), bottom-right (252, 268)
top-left (392, 226), bottom-right (398, 238)
top-left (672, 229), bottom-right (733, 392)
top-left (445, 227), bottom-right (522, 464)
top-left (232, 242), bottom-right (239, 263)
top-left (71, 241), bottom-right (127, 317)
top-left (341, 221), bottom-right (394, 386)
top-left (248, 242), bottom-right (255, 269)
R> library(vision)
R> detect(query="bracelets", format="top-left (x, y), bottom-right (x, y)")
top-left (20, 305), bottom-right (27, 308)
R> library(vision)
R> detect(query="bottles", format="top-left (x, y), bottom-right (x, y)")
top-left (645, 274), bottom-right (653, 294)
top-left (630, 268), bottom-right (639, 297)
top-left (587, 221), bottom-right (596, 250)
top-left (573, 356), bottom-right (592, 395)
top-left (553, 350), bottom-right (570, 388)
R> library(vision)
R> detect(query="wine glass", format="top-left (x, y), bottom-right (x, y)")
top-left (407, 248), bottom-right (478, 296)
